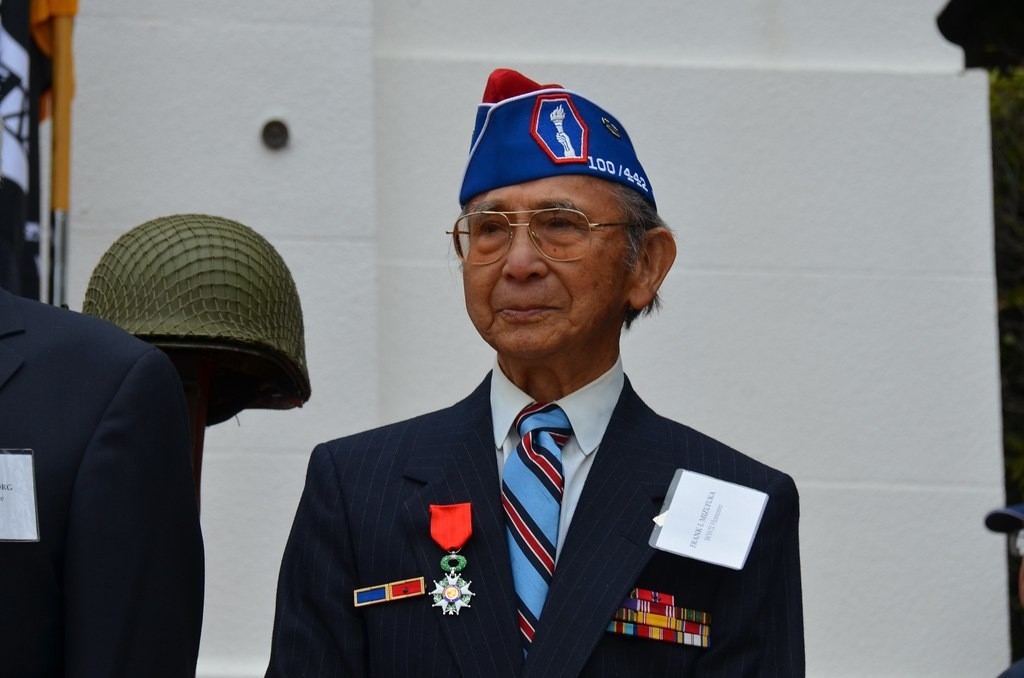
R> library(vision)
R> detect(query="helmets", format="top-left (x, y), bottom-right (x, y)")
top-left (82, 214), bottom-right (311, 426)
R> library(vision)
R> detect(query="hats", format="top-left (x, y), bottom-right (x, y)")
top-left (459, 68), bottom-right (658, 212)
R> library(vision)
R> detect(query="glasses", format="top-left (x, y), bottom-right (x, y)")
top-left (446, 209), bottom-right (627, 265)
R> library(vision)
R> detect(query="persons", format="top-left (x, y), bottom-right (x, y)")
top-left (263, 68), bottom-right (806, 678)
top-left (0, 286), bottom-right (205, 678)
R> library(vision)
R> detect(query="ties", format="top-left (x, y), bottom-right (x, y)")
top-left (502, 403), bottom-right (574, 663)
